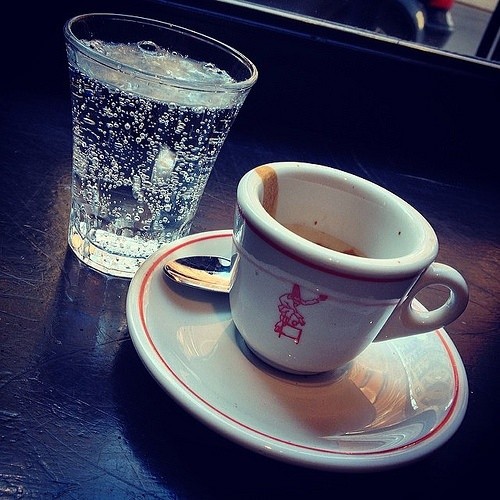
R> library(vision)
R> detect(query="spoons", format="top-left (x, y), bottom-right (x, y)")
top-left (162, 255), bottom-right (232, 294)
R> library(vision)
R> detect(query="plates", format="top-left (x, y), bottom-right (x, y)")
top-left (124, 227), bottom-right (469, 472)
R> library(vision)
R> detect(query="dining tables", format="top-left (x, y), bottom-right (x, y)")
top-left (0, 76), bottom-right (499, 500)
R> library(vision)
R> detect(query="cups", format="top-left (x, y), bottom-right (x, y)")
top-left (65, 12), bottom-right (259, 282)
top-left (228, 161), bottom-right (471, 376)
